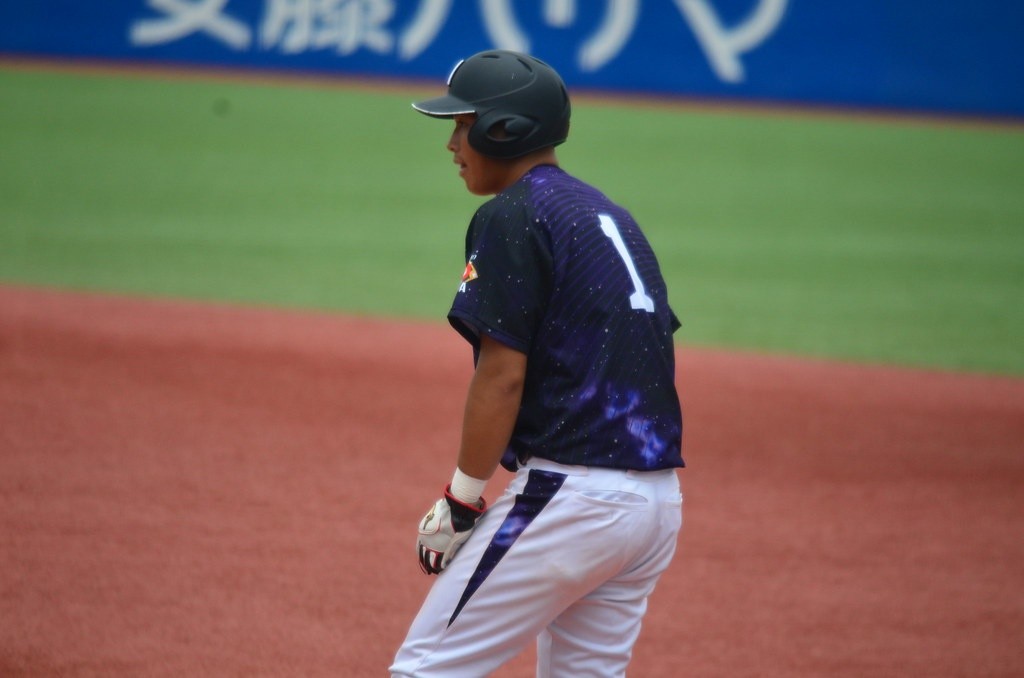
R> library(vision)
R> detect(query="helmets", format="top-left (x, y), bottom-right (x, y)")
top-left (413, 50), bottom-right (571, 159)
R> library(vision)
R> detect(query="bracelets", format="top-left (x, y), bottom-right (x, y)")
top-left (450, 467), bottom-right (488, 503)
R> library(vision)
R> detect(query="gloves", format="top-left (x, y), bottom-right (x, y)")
top-left (416, 483), bottom-right (486, 575)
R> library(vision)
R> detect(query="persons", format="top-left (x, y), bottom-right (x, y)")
top-left (389, 49), bottom-right (686, 678)
